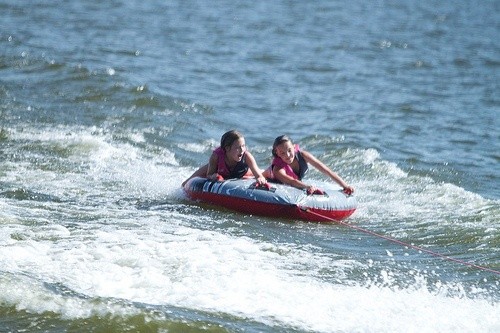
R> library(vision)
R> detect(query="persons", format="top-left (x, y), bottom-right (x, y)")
top-left (182, 130), bottom-right (267, 188)
top-left (262, 135), bottom-right (355, 195)
